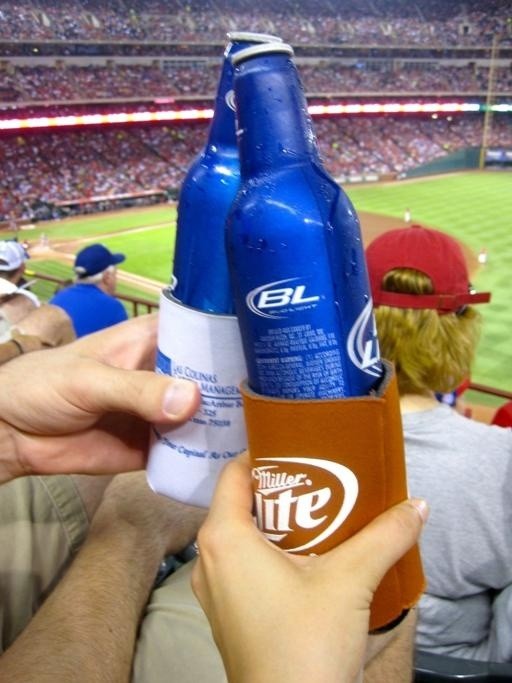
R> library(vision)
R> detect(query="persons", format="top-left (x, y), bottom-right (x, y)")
top-left (0, 224), bottom-right (512, 683)
top-left (0, 0), bottom-right (512, 229)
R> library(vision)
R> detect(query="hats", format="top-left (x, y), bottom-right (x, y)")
top-left (0, 241), bottom-right (30, 274)
top-left (364, 224), bottom-right (492, 314)
top-left (74, 241), bottom-right (125, 278)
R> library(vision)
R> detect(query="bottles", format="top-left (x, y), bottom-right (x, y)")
top-left (141, 25), bottom-right (290, 546)
top-left (226, 42), bottom-right (418, 634)
